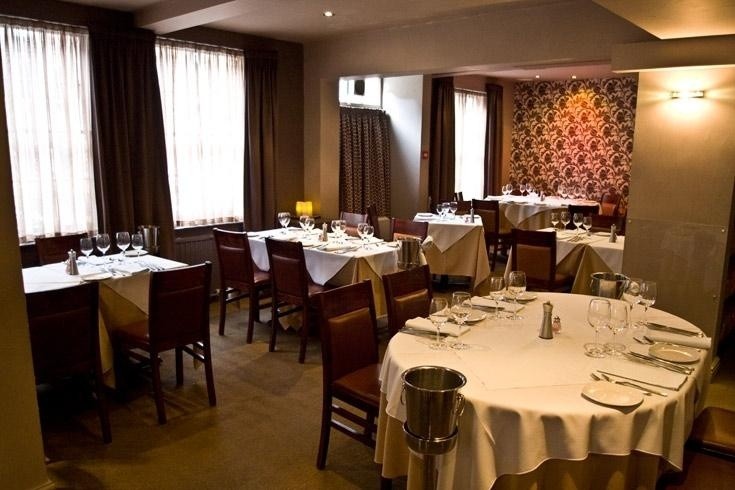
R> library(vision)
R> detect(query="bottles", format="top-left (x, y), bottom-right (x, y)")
top-left (539, 300), bottom-right (554, 339)
top-left (66, 248), bottom-right (79, 275)
top-left (469, 207), bottom-right (475, 223)
top-left (552, 315), bottom-right (561, 335)
top-left (610, 223), bottom-right (618, 243)
top-left (540, 190), bottom-right (546, 201)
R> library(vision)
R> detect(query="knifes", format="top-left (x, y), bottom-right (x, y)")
top-left (592, 370), bottom-right (668, 397)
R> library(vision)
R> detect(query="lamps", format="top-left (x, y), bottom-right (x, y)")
top-left (660, 89), bottom-right (708, 118)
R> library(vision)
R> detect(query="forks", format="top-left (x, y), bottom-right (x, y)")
top-left (630, 351), bottom-right (694, 376)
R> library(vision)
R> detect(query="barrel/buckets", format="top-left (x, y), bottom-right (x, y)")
top-left (396, 238), bottom-right (422, 270)
top-left (590, 272), bottom-right (630, 299)
top-left (400, 365), bottom-right (467, 440)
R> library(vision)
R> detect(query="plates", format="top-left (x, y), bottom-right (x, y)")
top-left (83, 272), bottom-right (114, 281)
top-left (510, 291), bottom-right (537, 301)
top-left (461, 307), bottom-right (486, 324)
top-left (647, 341), bottom-right (702, 364)
top-left (581, 379), bottom-right (645, 409)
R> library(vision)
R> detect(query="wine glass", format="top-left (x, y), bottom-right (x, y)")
top-left (277, 210), bottom-right (375, 248)
top-left (502, 183), bottom-right (539, 197)
top-left (428, 289), bottom-right (471, 352)
top-left (79, 230), bottom-right (170, 262)
top-left (582, 277), bottom-right (658, 357)
top-left (436, 201), bottom-right (458, 219)
top-left (550, 210), bottom-right (593, 238)
top-left (487, 270), bottom-right (528, 322)
top-left (558, 184), bottom-right (587, 202)
